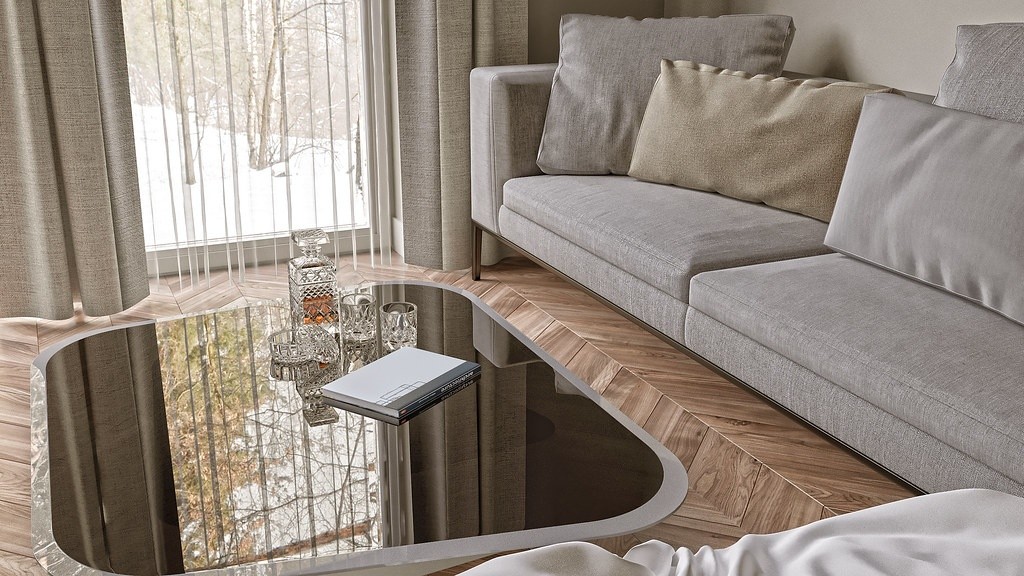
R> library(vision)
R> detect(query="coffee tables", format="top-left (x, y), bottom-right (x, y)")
top-left (29, 280), bottom-right (689, 576)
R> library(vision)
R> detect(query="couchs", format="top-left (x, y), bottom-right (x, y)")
top-left (469, 62), bottom-right (1024, 500)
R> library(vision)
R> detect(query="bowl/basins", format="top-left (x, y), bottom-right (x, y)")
top-left (270, 359), bottom-right (319, 381)
top-left (269, 328), bottom-right (309, 363)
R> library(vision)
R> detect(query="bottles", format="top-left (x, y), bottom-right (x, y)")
top-left (288, 229), bottom-right (339, 327)
top-left (296, 362), bottom-right (339, 427)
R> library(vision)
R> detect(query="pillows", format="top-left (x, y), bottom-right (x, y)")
top-left (932, 21), bottom-right (1024, 125)
top-left (823, 92), bottom-right (1024, 326)
top-left (536, 14), bottom-right (795, 176)
top-left (628, 59), bottom-right (905, 224)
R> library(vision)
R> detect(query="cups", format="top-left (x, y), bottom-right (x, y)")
top-left (379, 301), bottom-right (418, 351)
top-left (342, 342), bottom-right (377, 376)
top-left (339, 293), bottom-right (377, 341)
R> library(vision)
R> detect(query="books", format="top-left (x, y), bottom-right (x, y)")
top-left (320, 345), bottom-right (481, 417)
top-left (317, 372), bottom-right (482, 426)
